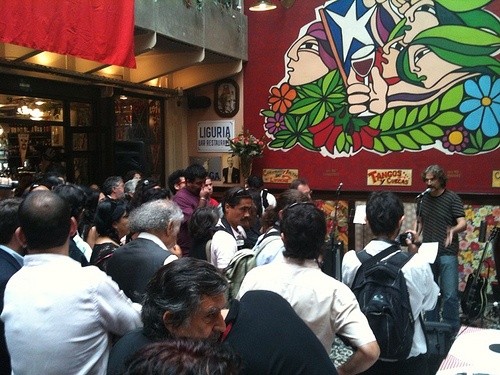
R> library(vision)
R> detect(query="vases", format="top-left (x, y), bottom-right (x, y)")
top-left (239, 152), bottom-right (250, 183)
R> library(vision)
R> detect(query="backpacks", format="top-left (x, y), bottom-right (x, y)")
top-left (220, 230), bottom-right (282, 310)
top-left (350, 250), bottom-right (420, 359)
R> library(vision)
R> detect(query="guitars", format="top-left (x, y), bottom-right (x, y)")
top-left (461, 229), bottom-right (499, 320)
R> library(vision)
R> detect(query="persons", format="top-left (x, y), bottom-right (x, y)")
top-left (405, 165), bottom-right (467, 326)
top-left (0, 164), bottom-right (440, 375)
top-left (222, 157), bottom-right (240, 184)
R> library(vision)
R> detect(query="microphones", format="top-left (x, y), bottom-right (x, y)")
top-left (416, 188), bottom-right (432, 198)
top-left (336, 183), bottom-right (343, 192)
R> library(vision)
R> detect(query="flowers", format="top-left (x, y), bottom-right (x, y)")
top-left (228, 131), bottom-right (266, 158)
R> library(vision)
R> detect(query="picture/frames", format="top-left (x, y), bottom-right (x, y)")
top-left (213, 78), bottom-right (240, 118)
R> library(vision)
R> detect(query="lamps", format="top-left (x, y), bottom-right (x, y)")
top-left (249, 0), bottom-right (277, 12)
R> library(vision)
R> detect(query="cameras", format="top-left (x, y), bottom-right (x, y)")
top-left (399, 231), bottom-right (414, 247)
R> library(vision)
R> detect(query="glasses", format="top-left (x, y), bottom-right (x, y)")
top-left (235, 188), bottom-right (249, 197)
